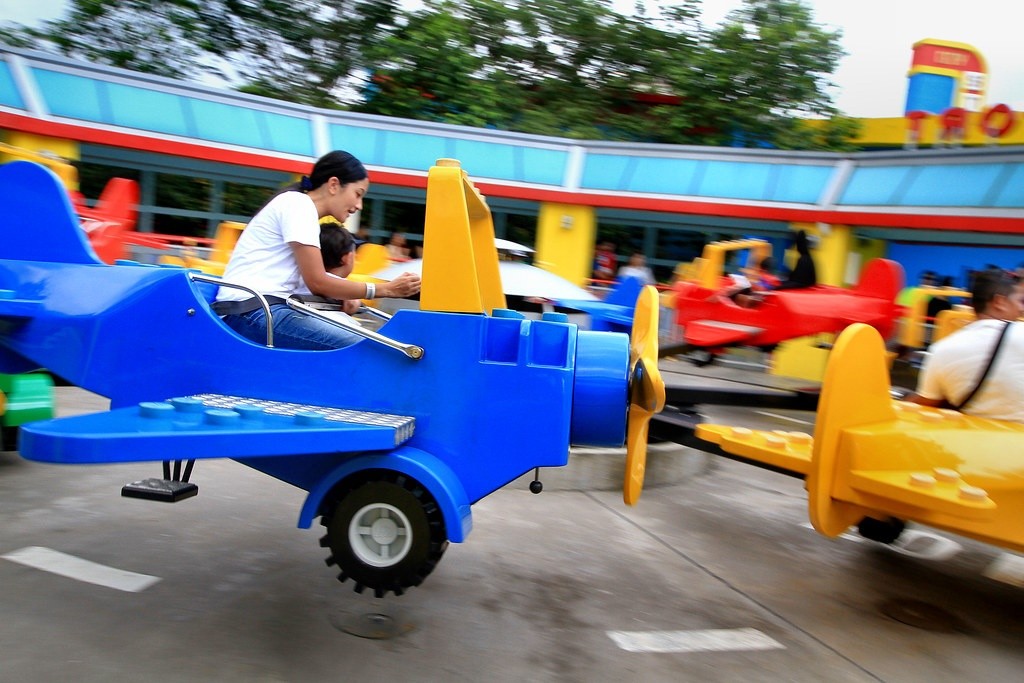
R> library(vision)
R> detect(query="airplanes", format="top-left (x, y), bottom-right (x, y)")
top-left (2, 159), bottom-right (665, 596)
top-left (651, 237), bottom-right (1023, 555)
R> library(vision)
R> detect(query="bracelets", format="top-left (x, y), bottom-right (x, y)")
top-left (366, 282), bottom-right (376, 301)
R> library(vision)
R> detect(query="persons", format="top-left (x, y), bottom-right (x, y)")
top-left (586, 237), bottom-right (655, 290)
top-left (905, 261), bottom-right (1023, 423)
top-left (724, 229), bottom-right (818, 313)
top-left (319, 224), bottom-right (366, 304)
top-left (217, 150), bottom-right (420, 352)
top-left (346, 221), bottom-right (423, 264)
